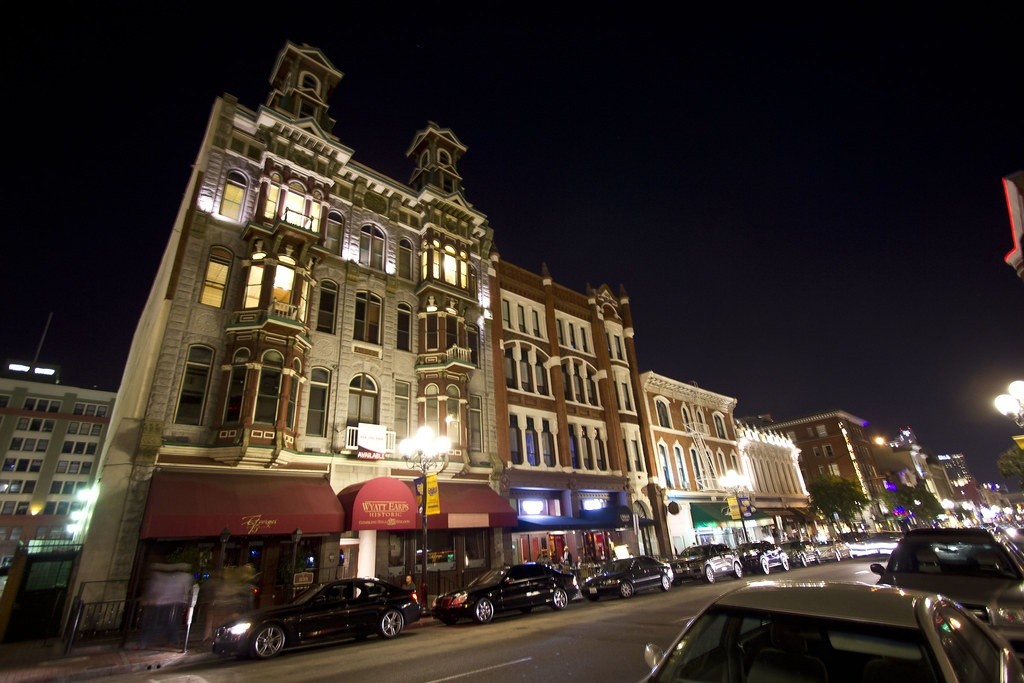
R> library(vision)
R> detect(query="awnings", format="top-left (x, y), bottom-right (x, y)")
top-left (138, 472), bottom-right (346, 539)
top-left (404, 481), bottom-right (518, 530)
top-left (690, 503), bottom-right (774, 527)
top-left (337, 477), bottom-right (416, 531)
top-left (513, 515), bottom-right (656, 532)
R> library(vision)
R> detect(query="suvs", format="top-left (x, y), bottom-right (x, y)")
top-left (870, 528), bottom-right (1024, 665)
top-left (735, 541), bottom-right (789, 575)
top-left (671, 542), bottom-right (744, 586)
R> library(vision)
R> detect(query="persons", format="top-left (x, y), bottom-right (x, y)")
top-left (563, 546), bottom-right (572, 566)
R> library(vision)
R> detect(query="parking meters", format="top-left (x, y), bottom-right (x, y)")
top-left (183, 583), bottom-right (200, 653)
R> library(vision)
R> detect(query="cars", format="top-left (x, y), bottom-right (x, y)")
top-left (212, 578), bottom-right (423, 660)
top-left (637, 580), bottom-right (1024, 683)
top-left (431, 562), bottom-right (580, 625)
top-left (779, 539), bottom-right (854, 568)
top-left (580, 555), bottom-right (674, 601)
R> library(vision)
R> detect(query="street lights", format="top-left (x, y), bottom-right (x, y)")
top-left (719, 469), bottom-right (749, 543)
top-left (399, 425), bottom-right (452, 618)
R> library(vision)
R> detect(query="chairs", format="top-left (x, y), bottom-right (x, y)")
top-left (746, 622), bottom-right (829, 683)
top-left (915, 547), bottom-right (942, 575)
top-left (862, 654), bottom-right (936, 683)
top-left (342, 588), bottom-right (348, 600)
top-left (328, 588), bottom-right (340, 602)
top-left (976, 550), bottom-right (997, 577)
